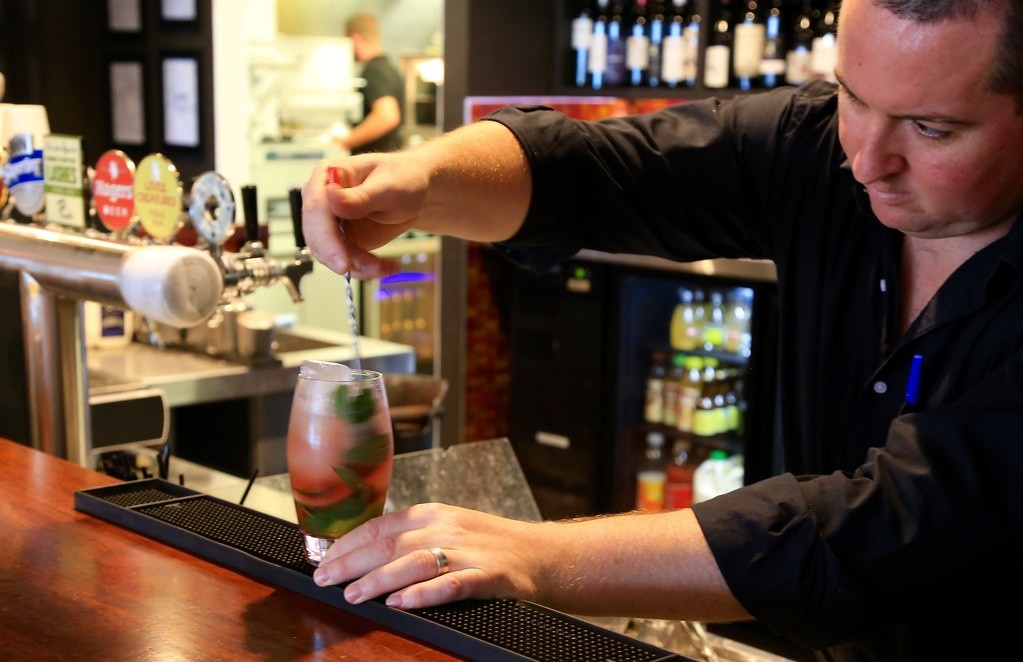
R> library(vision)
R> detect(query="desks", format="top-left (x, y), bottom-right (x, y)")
top-left (84, 327), bottom-right (415, 478)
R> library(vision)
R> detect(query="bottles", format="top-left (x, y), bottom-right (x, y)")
top-left (568, 0), bottom-right (843, 91)
top-left (637, 288), bottom-right (756, 514)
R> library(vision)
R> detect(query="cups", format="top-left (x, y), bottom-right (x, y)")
top-left (287, 368), bottom-right (394, 559)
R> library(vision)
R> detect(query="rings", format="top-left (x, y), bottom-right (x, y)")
top-left (430, 548), bottom-right (449, 576)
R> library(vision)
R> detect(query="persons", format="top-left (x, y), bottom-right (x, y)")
top-left (335, 10), bottom-right (407, 154)
top-left (301, 0), bottom-right (1023, 661)
